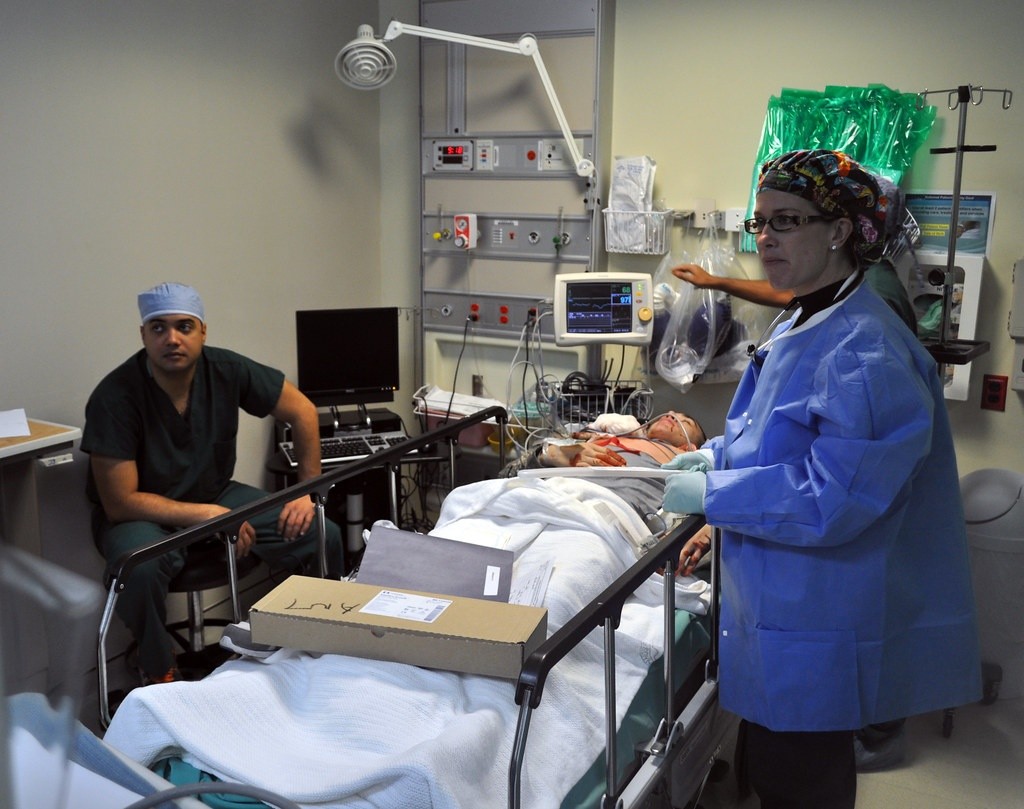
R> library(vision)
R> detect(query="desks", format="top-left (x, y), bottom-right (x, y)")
top-left (0, 409), bottom-right (81, 557)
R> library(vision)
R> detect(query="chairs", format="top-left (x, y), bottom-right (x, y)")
top-left (86, 468), bottom-right (243, 675)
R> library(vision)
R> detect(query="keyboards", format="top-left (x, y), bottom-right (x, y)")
top-left (280, 431), bottom-right (419, 465)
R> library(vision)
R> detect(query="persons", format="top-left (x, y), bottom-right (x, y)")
top-left (123, 411), bottom-right (707, 808)
top-left (78, 283), bottom-right (345, 689)
top-left (649, 145), bottom-right (988, 809)
top-left (669, 178), bottom-right (918, 773)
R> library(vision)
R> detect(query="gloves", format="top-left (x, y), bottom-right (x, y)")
top-left (660, 452), bottom-right (710, 474)
top-left (661, 471), bottom-right (706, 515)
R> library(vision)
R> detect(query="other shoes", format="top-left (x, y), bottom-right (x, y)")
top-left (138, 649), bottom-right (183, 687)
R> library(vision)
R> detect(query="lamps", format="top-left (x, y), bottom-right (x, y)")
top-left (335, 18), bottom-right (594, 178)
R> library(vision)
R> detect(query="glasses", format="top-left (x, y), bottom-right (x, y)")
top-left (744, 212), bottom-right (843, 235)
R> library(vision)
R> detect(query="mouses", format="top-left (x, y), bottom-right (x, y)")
top-left (419, 440), bottom-right (437, 454)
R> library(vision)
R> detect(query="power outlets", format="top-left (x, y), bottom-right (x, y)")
top-left (980, 373), bottom-right (1008, 411)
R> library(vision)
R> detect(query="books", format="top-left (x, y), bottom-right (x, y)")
top-left (356, 524), bottom-right (517, 603)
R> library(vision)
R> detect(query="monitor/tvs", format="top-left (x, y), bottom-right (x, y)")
top-left (566, 281), bottom-right (633, 334)
top-left (295, 307), bottom-right (399, 395)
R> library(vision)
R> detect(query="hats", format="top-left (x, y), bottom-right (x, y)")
top-left (137, 281), bottom-right (204, 326)
top-left (759, 148), bottom-right (888, 255)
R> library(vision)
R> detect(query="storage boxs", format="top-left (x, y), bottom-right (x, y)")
top-left (249, 576), bottom-right (547, 686)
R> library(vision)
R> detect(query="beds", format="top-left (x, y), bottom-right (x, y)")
top-left (80, 401), bottom-right (740, 806)
